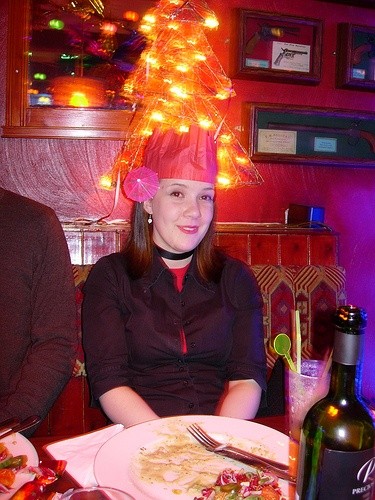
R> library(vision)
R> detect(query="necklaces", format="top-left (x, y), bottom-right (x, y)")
top-left (151, 241), bottom-right (196, 260)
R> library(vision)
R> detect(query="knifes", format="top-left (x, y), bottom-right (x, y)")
top-left (212, 449), bottom-right (289, 480)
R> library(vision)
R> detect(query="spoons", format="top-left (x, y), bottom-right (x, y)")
top-left (274, 333), bottom-right (295, 370)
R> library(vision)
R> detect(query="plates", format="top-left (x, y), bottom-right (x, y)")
top-left (93, 414), bottom-right (291, 500)
top-left (0, 428), bottom-right (39, 500)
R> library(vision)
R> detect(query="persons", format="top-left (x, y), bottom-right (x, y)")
top-left (80, 125), bottom-right (267, 429)
top-left (0, 188), bottom-right (78, 437)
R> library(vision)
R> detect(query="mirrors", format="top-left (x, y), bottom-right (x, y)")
top-left (0, 1), bottom-right (152, 141)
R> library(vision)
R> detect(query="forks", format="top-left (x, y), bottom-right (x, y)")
top-left (186, 421), bottom-right (290, 470)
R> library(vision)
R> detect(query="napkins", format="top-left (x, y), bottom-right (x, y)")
top-left (42, 423), bottom-right (126, 488)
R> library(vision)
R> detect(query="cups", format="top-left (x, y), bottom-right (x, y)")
top-left (58, 486), bottom-right (135, 500)
top-left (287, 357), bottom-right (331, 500)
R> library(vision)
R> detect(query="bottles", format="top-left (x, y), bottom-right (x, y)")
top-left (293, 304), bottom-right (375, 500)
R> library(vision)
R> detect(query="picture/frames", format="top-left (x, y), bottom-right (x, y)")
top-left (337, 21), bottom-right (375, 92)
top-left (249, 102), bottom-right (375, 168)
top-left (232, 7), bottom-right (323, 85)
